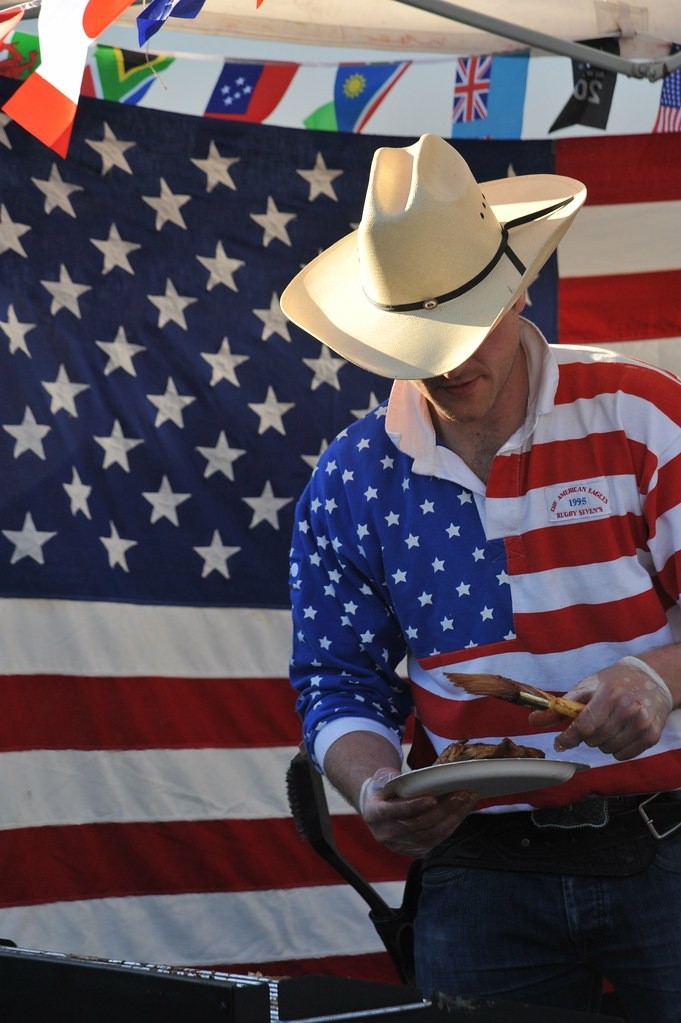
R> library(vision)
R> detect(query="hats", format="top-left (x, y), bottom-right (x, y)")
top-left (279, 132), bottom-right (588, 380)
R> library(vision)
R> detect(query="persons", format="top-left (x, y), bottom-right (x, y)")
top-left (280, 132), bottom-right (681, 1023)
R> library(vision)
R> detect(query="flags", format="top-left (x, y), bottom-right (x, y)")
top-left (80, 43), bottom-right (176, 107)
top-left (333, 61), bottom-right (412, 132)
top-left (204, 63), bottom-right (300, 124)
top-left (547, 37), bottom-right (621, 134)
top-left (0, 30), bottom-right (41, 81)
top-left (1, 0), bottom-right (136, 161)
top-left (0, 76), bottom-right (681, 987)
top-left (652, 43), bottom-right (681, 133)
top-left (450, 55), bottom-right (494, 122)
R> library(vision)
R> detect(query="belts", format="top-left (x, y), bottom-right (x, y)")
top-left (418, 790), bottom-right (681, 861)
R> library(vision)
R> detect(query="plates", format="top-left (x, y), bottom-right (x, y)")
top-left (383, 760), bottom-right (591, 801)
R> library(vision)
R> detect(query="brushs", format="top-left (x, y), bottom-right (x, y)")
top-left (442, 671), bottom-right (583, 719)
